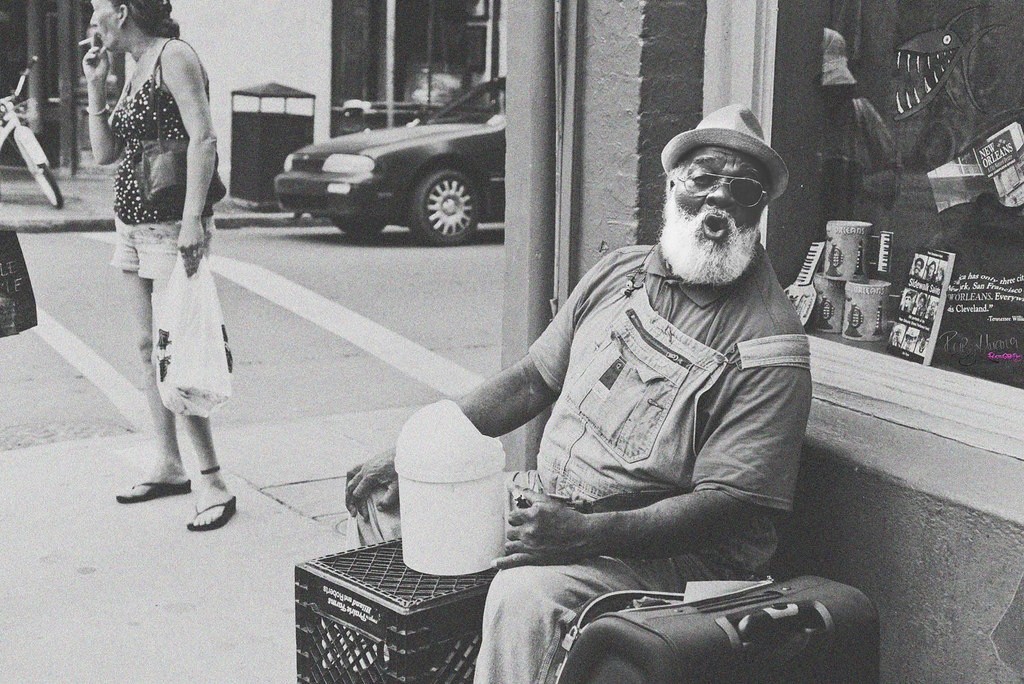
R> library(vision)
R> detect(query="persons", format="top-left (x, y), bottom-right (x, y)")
top-left (82, 0), bottom-right (237, 533)
top-left (817, 27), bottom-right (905, 242)
top-left (344, 103), bottom-right (814, 684)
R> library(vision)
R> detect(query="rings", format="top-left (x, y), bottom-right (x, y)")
top-left (192, 250), bottom-right (198, 258)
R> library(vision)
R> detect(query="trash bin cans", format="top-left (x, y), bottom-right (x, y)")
top-left (230, 83), bottom-right (317, 210)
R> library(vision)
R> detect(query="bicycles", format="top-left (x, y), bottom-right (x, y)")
top-left (0, 53), bottom-right (64, 209)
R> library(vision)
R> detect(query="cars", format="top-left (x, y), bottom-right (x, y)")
top-left (274, 76), bottom-right (506, 247)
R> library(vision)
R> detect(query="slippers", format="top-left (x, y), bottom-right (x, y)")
top-left (115, 479), bottom-right (192, 504)
top-left (187, 496), bottom-right (237, 530)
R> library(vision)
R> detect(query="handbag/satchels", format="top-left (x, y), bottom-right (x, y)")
top-left (131, 38), bottom-right (226, 212)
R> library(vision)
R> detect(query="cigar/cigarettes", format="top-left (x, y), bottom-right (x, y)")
top-left (79, 36), bottom-right (93, 46)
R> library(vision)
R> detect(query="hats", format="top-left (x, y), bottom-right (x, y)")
top-left (823, 27), bottom-right (856, 86)
top-left (659, 104), bottom-right (789, 203)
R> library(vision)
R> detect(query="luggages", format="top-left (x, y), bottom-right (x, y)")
top-left (552, 573), bottom-right (880, 683)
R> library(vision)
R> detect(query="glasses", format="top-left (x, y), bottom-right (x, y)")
top-left (675, 172), bottom-right (768, 207)
